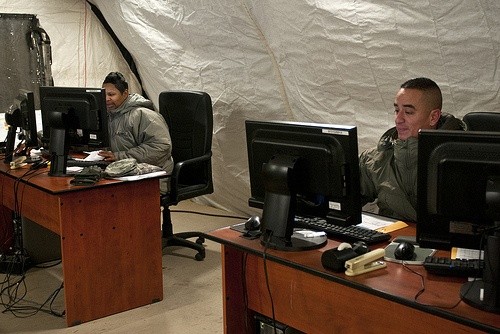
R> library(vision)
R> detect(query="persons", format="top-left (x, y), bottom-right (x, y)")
top-left (98, 72), bottom-right (174, 196)
top-left (358, 77), bottom-right (465, 222)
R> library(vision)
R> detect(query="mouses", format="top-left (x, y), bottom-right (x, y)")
top-left (395, 241), bottom-right (415, 262)
top-left (246, 215), bottom-right (261, 230)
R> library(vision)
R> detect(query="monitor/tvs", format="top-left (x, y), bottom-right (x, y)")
top-left (413, 128), bottom-right (500, 314)
top-left (244, 119), bottom-right (362, 251)
top-left (39, 85), bottom-right (109, 177)
top-left (5, 88), bottom-right (38, 164)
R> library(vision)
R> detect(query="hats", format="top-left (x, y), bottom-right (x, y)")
top-left (105, 158), bottom-right (144, 177)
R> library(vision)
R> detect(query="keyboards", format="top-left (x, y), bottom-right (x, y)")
top-left (423, 255), bottom-right (485, 278)
top-left (293, 213), bottom-right (391, 245)
top-left (67, 159), bottom-right (112, 169)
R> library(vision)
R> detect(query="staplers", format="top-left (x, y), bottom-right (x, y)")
top-left (345, 248), bottom-right (386, 276)
top-left (10, 156), bottom-right (27, 169)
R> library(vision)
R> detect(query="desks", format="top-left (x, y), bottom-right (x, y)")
top-left (202, 210), bottom-right (500, 334)
top-left (0, 150), bottom-right (172, 327)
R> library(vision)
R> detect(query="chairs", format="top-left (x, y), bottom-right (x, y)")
top-left (159, 91), bottom-right (214, 260)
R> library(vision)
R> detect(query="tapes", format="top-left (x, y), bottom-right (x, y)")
top-left (337, 242), bottom-right (352, 251)
